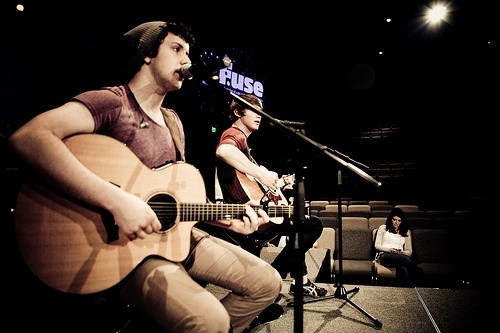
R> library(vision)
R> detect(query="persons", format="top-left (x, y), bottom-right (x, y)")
top-left (9, 21), bottom-right (283, 333)
top-left (375, 208), bottom-right (427, 287)
top-left (215, 95), bottom-right (327, 298)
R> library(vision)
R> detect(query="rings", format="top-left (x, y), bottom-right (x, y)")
top-left (253, 226), bottom-right (258, 230)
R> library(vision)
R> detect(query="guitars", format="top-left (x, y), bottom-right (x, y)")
top-left (216, 164), bottom-right (296, 235)
top-left (11, 133), bottom-right (312, 294)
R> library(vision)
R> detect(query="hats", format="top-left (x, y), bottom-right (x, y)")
top-left (123, 20), bottom-right (171, 73)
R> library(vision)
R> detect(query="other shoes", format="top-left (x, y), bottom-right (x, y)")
top-left (289, 278), bottom-right (327, 298)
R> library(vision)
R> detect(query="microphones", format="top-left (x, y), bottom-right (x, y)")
top-left (179, 57), bottom-right (232, 78)
top-left (270, 118), bottom-right (305, 126)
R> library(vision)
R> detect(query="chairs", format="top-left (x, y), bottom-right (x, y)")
top-left (310, 200), bottom-right (472, 283)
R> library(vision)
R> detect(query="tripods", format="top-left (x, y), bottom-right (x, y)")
top-left (287, 145), bottom-right (385, 328)
